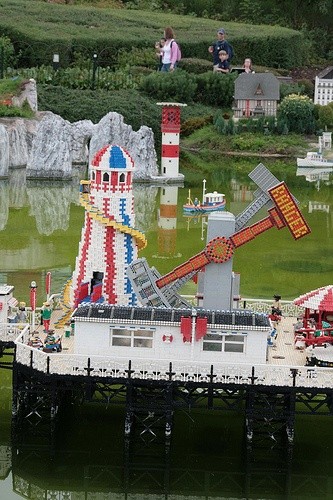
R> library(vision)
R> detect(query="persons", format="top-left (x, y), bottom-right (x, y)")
top-left (208, 28), bottom-right (232, 69)
top-left (212, 50), bottom-right (230, 74)
top-left (41, 302), bottom-right (51, 333)
top-left (156, 38), bottom-right (167, 72)
top-left (267, 295), bottom-right (282, 347)
top-left (17, 301), bottom-right (28, 323)
top-left (155, 28), bottom-right (178, 73)
top-left (242, 58), bottom-right (255, 74)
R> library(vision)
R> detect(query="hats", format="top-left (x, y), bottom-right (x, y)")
top-left (219, 28), bottom-right (224, 35)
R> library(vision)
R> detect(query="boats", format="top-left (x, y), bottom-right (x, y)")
top-left (296, 152), bottom-right (333, 168)
top-left (296, 166), bottom-right (333, 192)
top-left (182, 179), bottom-right (227, 217)
